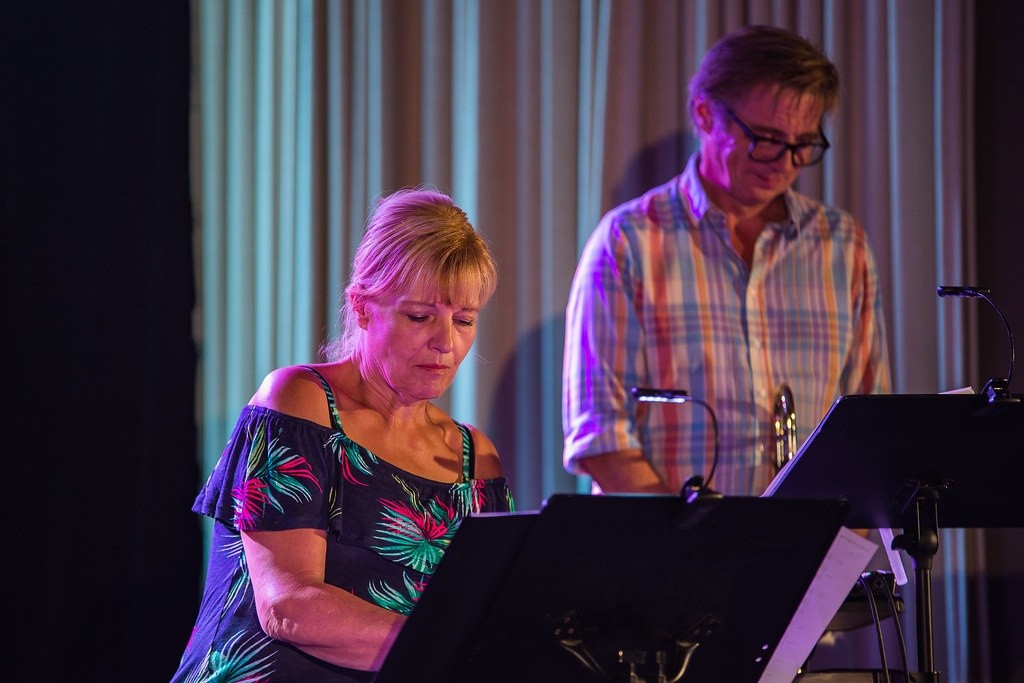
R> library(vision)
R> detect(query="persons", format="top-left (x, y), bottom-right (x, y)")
top-left (170, 190), bottom-right (514, 683)
top-left (562, 25), bottom-right (894, 538)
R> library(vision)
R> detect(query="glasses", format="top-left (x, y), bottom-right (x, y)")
top-left (717, 99), bottom-right (830, 168)
